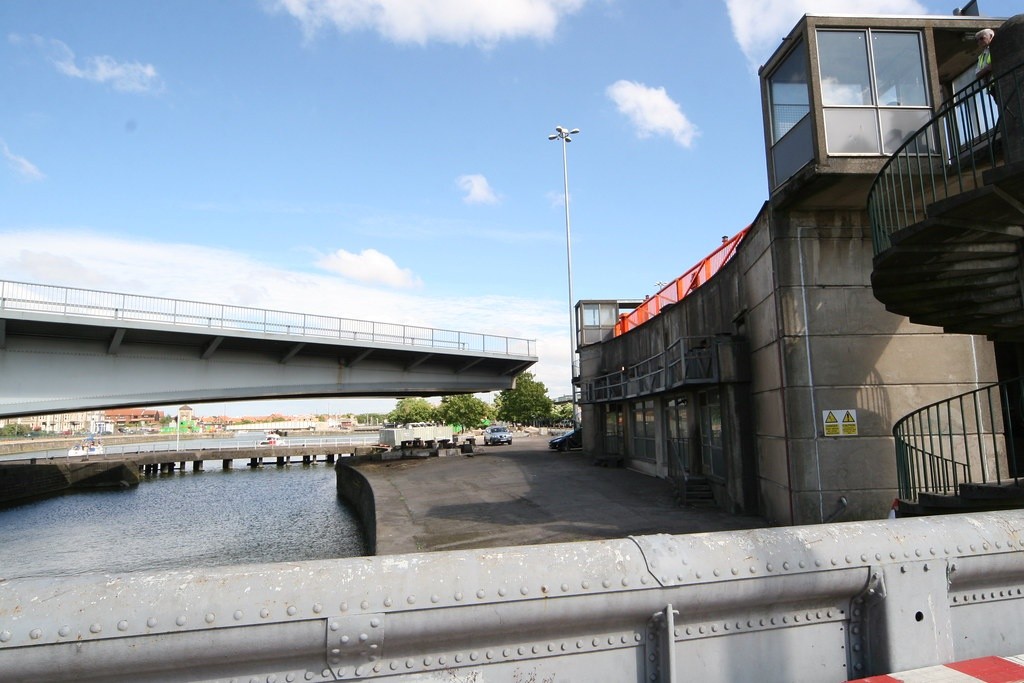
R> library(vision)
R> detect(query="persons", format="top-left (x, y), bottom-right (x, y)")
top-left (974, 28), bottom-right (997, 106)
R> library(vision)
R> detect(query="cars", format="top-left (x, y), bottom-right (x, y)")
top-left (548, 427), bottom-right (583, 452)
top-left (484, 426), bottom-right (512, 446)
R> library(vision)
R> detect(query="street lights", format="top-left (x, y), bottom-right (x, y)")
top-left (548, 125), bottom-right (580, 431)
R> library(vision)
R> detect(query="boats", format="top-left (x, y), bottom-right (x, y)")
top-left (256, 433), bottom-right (286, 447)
top-left (68, 435), bottom-right (105, 456)
